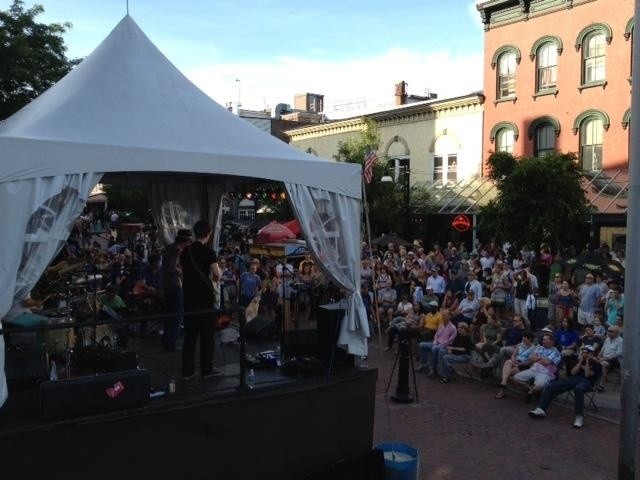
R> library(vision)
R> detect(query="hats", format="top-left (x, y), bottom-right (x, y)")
top-left (175, 229), bottom-right (192, 242)
top-left (429, 301), bottom-right (438, 307)
top-left (426, 285), bottom-right (432, 290)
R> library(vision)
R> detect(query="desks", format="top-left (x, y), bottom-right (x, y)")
top-left (0, 304), bottom-right (250, 395)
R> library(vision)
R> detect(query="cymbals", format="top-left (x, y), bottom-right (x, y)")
top-left (72, 274), bottom-right (102, 283)
top-left (55, 284), bottom-right (88, 290)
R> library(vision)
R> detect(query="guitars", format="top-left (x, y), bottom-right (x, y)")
top-left (208, 270), bottom-right (221, 304)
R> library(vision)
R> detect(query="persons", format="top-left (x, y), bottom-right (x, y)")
top-left (4, 209), bottom-right (326, 379)
top-left (359, 241), bottom-right (624, 427)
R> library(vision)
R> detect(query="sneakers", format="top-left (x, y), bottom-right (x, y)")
top-left (203, 370), bottom-right (224, 379)
top-left (183, 371), bottom-right (197, 381)
top-left (573, 415), bottom-right (583, 427)
top-left (528, 408), bottom-right (546, 417)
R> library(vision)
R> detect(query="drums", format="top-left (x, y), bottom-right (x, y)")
top-left (39, 288), bottom-right (121, 355)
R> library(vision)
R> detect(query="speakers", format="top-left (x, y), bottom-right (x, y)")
top-left (317, 304), bottom-right (355, 376)
top-left (280, 330), bottom-right (317, 354)
top-left (81, 349), bottom-right (137, 373)
top-left (247, 317), bottom-right (281, 341)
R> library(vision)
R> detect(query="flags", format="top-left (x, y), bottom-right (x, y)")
top-left (364, 144), bottom-right (379, 184)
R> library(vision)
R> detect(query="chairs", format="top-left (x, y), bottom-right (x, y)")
top-left (407, 323), bottom-right (606, 415)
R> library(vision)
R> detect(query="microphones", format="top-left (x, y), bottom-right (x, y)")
top-left (260, 246), bottom-right (271, 251)
